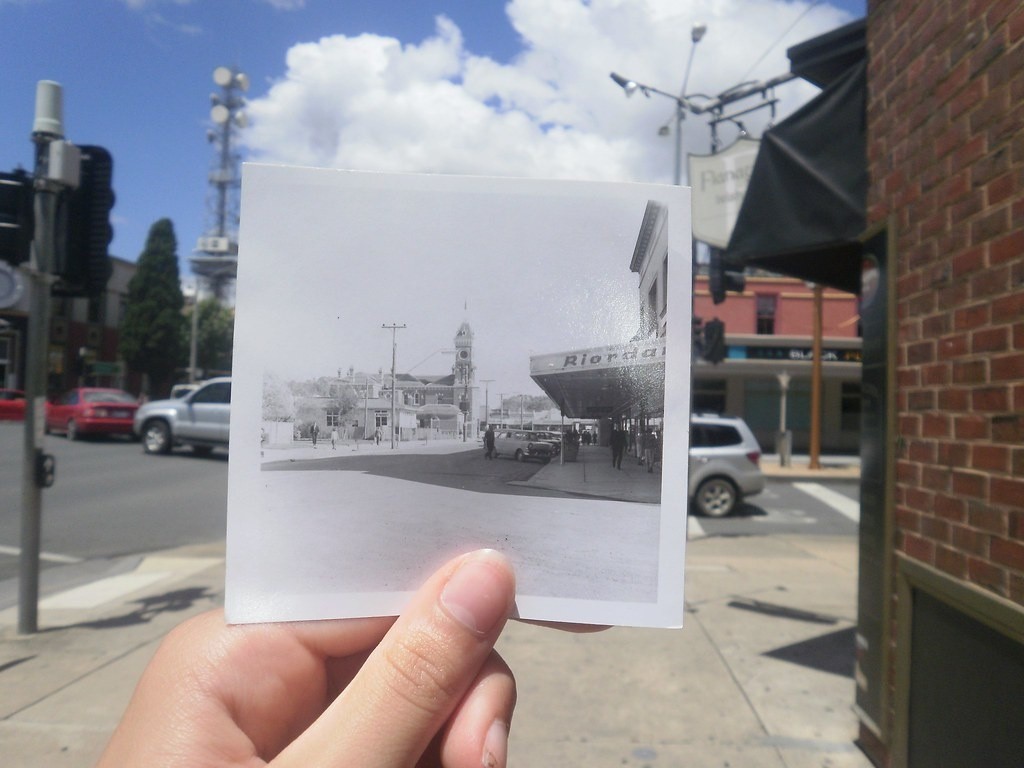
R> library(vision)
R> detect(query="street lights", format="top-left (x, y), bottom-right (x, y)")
top-left (660, 93), bottom-right (717, 154)
top-left (672, 20), bottom-right (706, 184)
top-left (392, 346), bottom-right (458, 449)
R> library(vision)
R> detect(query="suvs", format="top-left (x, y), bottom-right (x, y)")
top-left (130, 375), bottom-right (231, 454)
top-left (688, 415), bottom-right (765, 518)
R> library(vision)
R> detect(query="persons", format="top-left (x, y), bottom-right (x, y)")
top-left (593, 431), bottom-right (597, 446)
top-left (568, 429), bottom-right (580, 441)
top-left (643, 428), bottom-right (656, 472)
top-left (581, 431), bottom-right (591, 445)
top-left (375, 427), bottom-right (382, 446)
top-left (636, 434), bottom-right (645, 465)
top-left (610, 422), bottom-right (626, 469)
top-left (483, 425), bottom-right (495, 460)
top-left (330, 428), bottom-right (339, 450)
top-left (310, 422), bottom-right (319, 446)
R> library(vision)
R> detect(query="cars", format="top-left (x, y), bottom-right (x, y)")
top-left (45, 388), bottom-right (140, 442)
top-left (524, 431), bottom-right (564, 454)
top-left (482, 430), bottom-right (553, 462)
top-left (1, 389), bottom-right (50, 422)
top-left (170, 385), bottom-right (197, 401)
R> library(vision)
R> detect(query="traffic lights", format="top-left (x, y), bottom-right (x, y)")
top-left (692, 316), bottom-right (703, 354)
top-left (0, 168), bottom-right (31, 265)
top-left (709, 248), bottom-right (746, 304)
